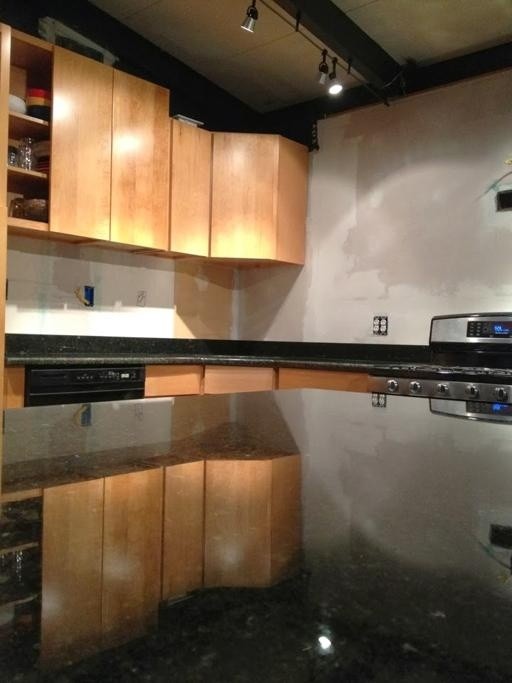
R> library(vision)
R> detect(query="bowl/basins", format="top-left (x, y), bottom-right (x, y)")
top-left (8, 88), bottom-right (51, 123)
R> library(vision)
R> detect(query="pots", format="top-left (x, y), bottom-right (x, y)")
top-left (14, 193), bottom-right (48, 222)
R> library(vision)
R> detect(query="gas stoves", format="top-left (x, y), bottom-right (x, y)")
top-left (369, 360), bottom-right (512, 406)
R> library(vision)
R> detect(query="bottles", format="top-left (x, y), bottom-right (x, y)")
top-left (9, 137), bottom-right (32, 170)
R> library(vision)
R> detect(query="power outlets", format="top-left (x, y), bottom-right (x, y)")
top-left (372, 313), bottom-right (390, 337)
top-left (370, 391), bottom-right (386, 408)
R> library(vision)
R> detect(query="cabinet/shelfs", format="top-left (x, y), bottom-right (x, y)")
top-left (103, 468), bottom-right (163, 631)
top-left (201, 364), bottom-right (276, 393)
top-left (0, 490), bottom-right (44, 671)
top-left (276, 135), bottom-right (308, 267)
top-left (170, 117), bottom-right (210, 259)
top-left (112, 69), bottom-right (169, 250)
top-left (145, 362), bottom-right (204, 397)
top-left (164, 460), bottom-right (204, 599)
top-left (270, 453), bottom-right (300, 581)
top-left (277, 365), bottom-right (369, 392)
top-left (4, 28), bottom-right (110, 240)
top-left (3, 361), bottom-right (23, 408)
top-left (45, 476), bottom-right (105, 657)
top-left (205, 456), bottom-right (270, 589)
top-left (210, 129), bottom-right (278, 262)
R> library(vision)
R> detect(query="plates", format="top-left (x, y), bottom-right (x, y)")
top-left (31, 141), bottom-right (49, 174)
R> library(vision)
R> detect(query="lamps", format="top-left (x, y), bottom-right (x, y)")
top-left (239, 0), bottom-right (344, 96)
